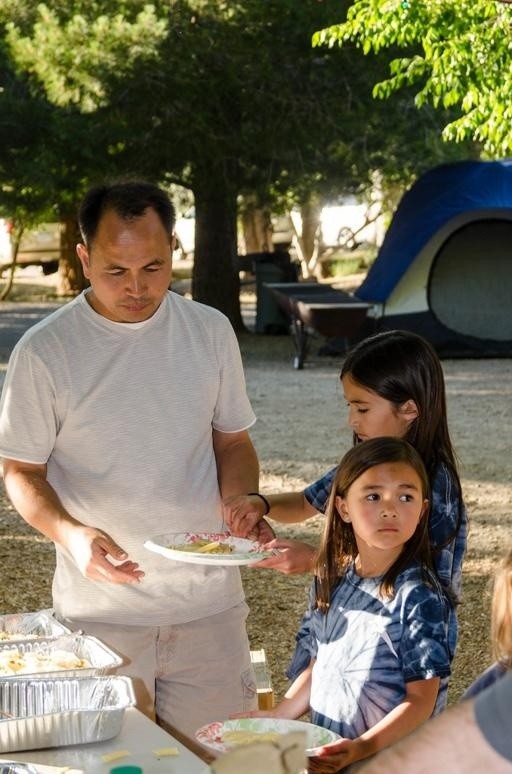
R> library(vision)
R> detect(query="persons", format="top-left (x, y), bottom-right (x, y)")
top-left (228, 439), bottom-right (462, 774)
top-left (225, 329), bottom-right (468, 718)
top-left (0, 183), bottom-right (276, 757)
top-left (345, 544), bottom-right (512, 774)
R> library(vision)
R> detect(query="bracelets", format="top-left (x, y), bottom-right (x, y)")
top-left (248, 493), bottom-right (270, 515)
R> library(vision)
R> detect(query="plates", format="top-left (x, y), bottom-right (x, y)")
top-left (195, 719), bottom-right (342, 761)
top-left (144, 530), bottom-right (274, 566)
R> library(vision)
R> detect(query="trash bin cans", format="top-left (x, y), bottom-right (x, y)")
top-left (252, 262), bottom-right (301, 335)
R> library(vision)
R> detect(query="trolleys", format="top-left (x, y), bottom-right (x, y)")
top-left (263, 272), bottom-right (378, 371)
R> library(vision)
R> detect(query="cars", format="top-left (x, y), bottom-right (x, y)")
top-left (0, 213), bottom-right (65, 276)
top-left (290, 171), bottom-right (393, 252)
top-left (167, 179), bottom-right (293, 267)
